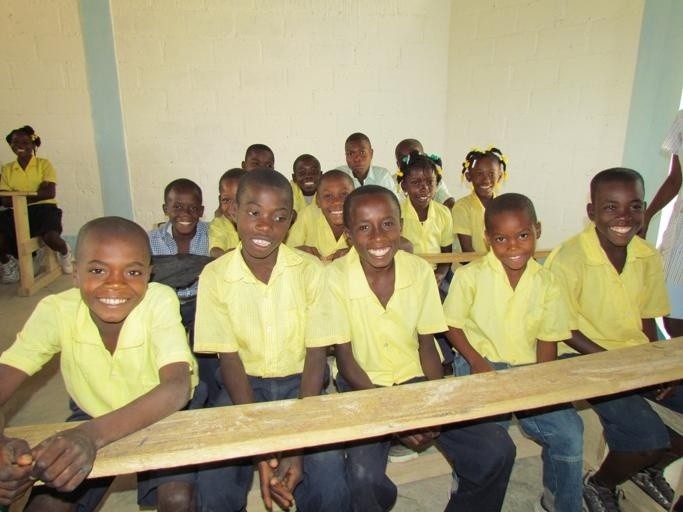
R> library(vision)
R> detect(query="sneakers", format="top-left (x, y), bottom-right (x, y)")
top-left (630, 467), bottom-right (675, 512)
top-left (540, 492), bottom-right (587, 512)
top-left (60, 242), bottom-right (73, 273)
top-left (0, 254), bottom-right (20, 283)
top-left (583, 470), bottom-right (622, 512)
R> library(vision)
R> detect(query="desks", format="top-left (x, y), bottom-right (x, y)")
top-left (0, 185), bottom-right (62, 299)
top-left (412, 244), bottom-right (556, 266)
top-left (0, 327), bottom-right (683, 510)
